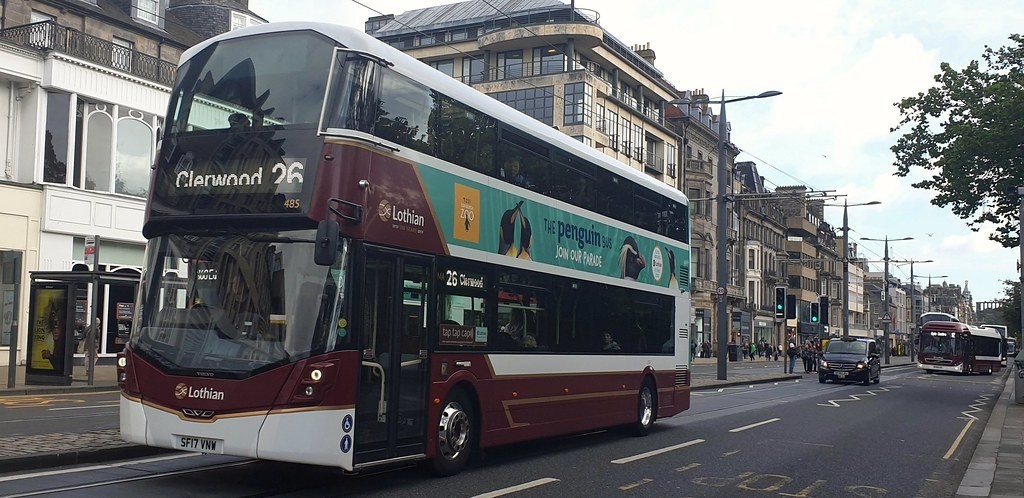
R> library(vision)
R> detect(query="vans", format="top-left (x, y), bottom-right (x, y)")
top-left (818, 337), bottom-right (881, 386)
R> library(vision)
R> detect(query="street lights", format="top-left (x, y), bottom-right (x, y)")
top-left (860, 235), bottom-right (914, 364)
top-left (815, 201), bottom-right (883, 337)
top-left (914, 273), bottom-right (949, 312)
top-left (667, 90), bottom-right (782, 380)
top-left (891, 259), bottom-right (934, 363)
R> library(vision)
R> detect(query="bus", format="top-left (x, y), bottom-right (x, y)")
top-left (116, 21), bottom-right (694, 474)
top-left (1007, 337), bottom-right (1017, 357)
top-left (977, 324), bottom-right (1008, 368)
top-left (916, 320), bottom-right (1002, 375)
top-left (919, 312), bottom-right (960, 335)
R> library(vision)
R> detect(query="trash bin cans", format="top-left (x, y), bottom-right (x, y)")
top-left (1014, 359), bottom-right (1024, 404)
top-left (728, 345), bottom-right (743, 361)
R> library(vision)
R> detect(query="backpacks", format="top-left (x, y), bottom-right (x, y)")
top-left (787, 349), bottom-right (794, 356)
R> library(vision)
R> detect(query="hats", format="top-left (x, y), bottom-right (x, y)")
top-left (790, 343), bottom-right (794, 348)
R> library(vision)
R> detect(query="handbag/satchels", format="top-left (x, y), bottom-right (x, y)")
top-left (77, 340), bottom-right (87, 354)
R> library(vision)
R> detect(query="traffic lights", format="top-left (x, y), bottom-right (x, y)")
top-left (810, 302), bottom-right (821, 324)
top-left (775, 287), bottom-right (785, 321)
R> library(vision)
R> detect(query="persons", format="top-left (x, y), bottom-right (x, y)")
top-left (926, 342), bottom-right (938, 352)
top-left (220, 273), bottom-right (269, 340)
top-left (479, 144), bottom-right (493, 171)
top-left (691, 339), bottom-right (697, 361)
top-left (505, 308), bottom-right (536, 346)
top-left (889, 346), bottom-right (898, 357)
top-left (741, 340), bottom-right (783, 362)
top-left (699, 340), bottom-right (712, 358)
top-left (508, 159), bottom-right (530, 186)
top-left (602, 331), bottom-right (620, 350)
top-left (787, 337), bottom-right (817, 374)
top-left (80, 317), bottom-right (101, 374)
